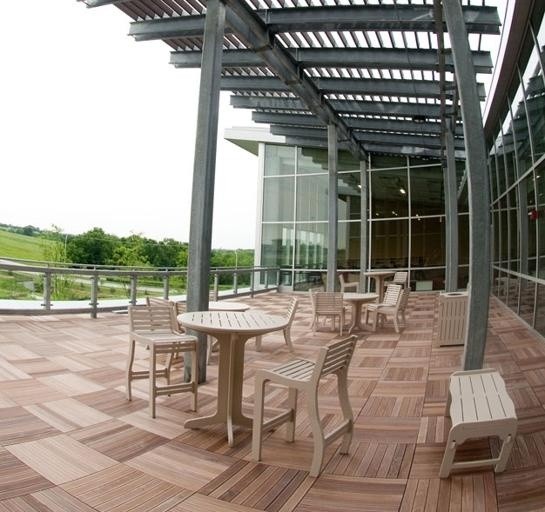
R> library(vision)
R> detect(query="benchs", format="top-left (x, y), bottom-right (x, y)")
top-left (437, 367), bottom-right (516, 478)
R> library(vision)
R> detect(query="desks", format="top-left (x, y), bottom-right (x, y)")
top-left (204, 300), bottom-right (251, 312)
top-left (170, 308), bottom-right (289, 442)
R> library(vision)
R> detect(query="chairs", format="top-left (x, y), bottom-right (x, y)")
top-left (256, 298), bottom-right (300, 354)
top-left (250, 333), bottom-right (362, 481)
top-left (310, 269), bottom-right (414, 333)
top-left (143, 295), bottom-right (187, 361)
top-left (121, 303), bottom-right (203, 419)
